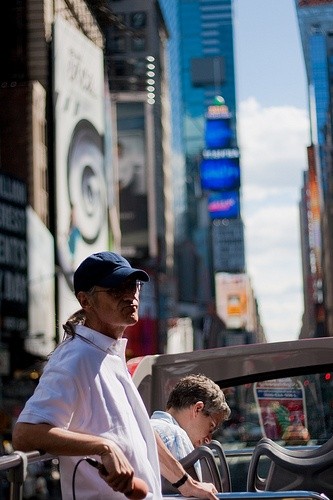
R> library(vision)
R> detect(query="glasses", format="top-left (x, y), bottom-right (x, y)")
top-left (204, 407), bottom-right (220, 434)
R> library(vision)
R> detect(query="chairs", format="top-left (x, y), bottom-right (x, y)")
top-left (245, 436), bottom-right (332, 493)
top-left (157, 438), bottom-right (231, 493)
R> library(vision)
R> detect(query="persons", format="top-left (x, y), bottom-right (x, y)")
top-left (149, 372), bottom-right (232, 482)
top-left (14, 251), bottom-right (220, 500)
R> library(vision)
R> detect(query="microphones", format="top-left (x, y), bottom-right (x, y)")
top-left (86, 456), bottom-right (148, 500)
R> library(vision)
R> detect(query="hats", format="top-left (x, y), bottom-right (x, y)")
top-left (73, 251), bottom-right (150, 292)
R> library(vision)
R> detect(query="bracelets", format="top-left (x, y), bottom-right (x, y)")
top-left (171, 472), bottom-right (189, 489)
